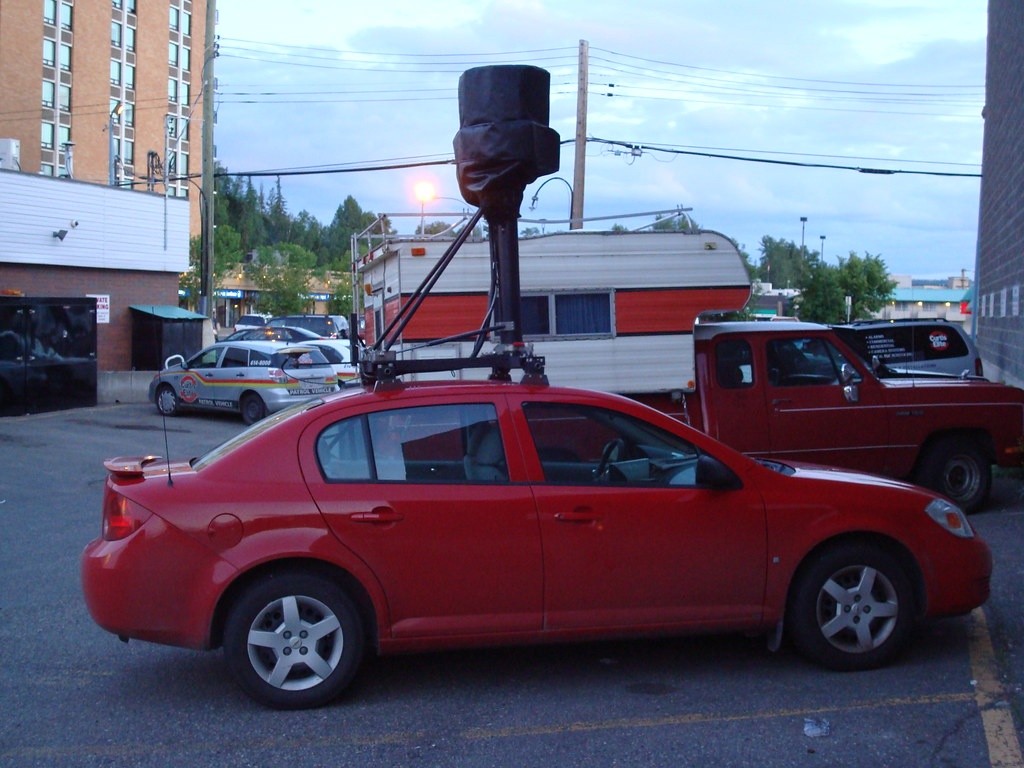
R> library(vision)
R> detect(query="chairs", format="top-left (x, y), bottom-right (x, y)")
top-left (478, 430), bottom-right (500, 482)
top-left (463, 418), bottom-right (486, 480)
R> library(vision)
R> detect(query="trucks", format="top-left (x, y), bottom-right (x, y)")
top-left (349, 211), bottom-right (1023, 517)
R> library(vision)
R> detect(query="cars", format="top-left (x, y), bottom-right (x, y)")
top-left (146, 308), bottom-right (361, 426)
top-left (80, 352), bottom-right (994, 710)
top-left (1, 321), bottom-right (100, 404)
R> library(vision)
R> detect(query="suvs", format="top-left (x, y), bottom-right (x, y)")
top-left (761, 317), bottom-right (985, 384)
